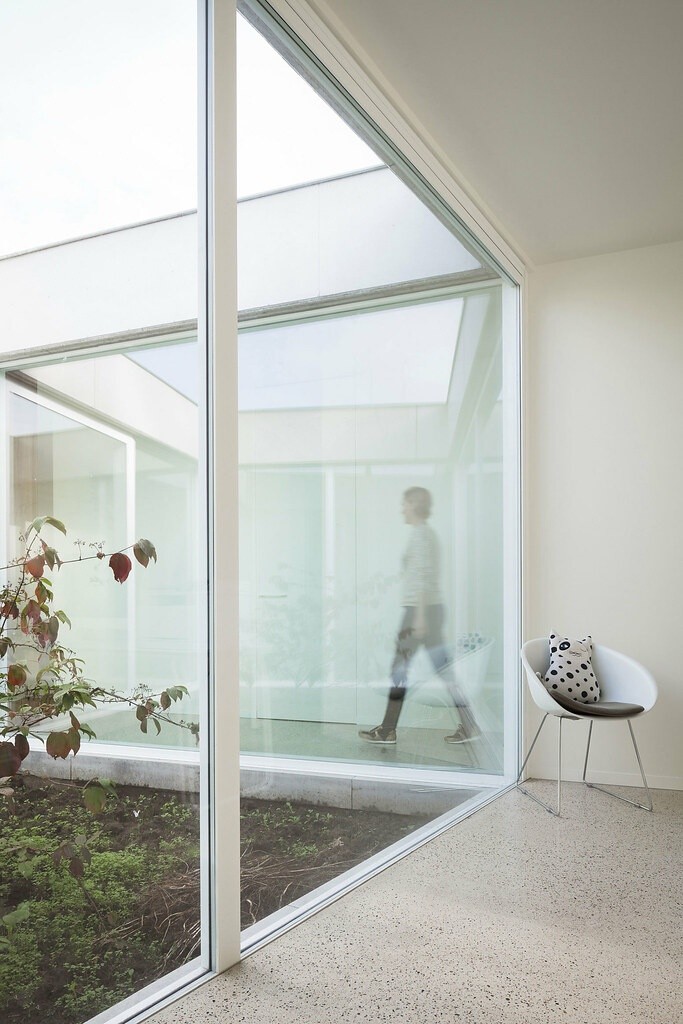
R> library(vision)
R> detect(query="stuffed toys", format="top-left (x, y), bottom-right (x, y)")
top-left (536, 628), bottom-right (601, 703)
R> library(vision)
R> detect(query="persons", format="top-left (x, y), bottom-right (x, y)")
top-left (358, 486), bottom-right (482, 744)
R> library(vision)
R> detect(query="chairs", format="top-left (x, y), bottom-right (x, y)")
top-left (516, 637), bottom-right (659, 817)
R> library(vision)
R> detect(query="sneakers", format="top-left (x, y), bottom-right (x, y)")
top-left (444, 723), bottom-right (483, 743)
top-left (358, 725), bottom-right (397, 744)
